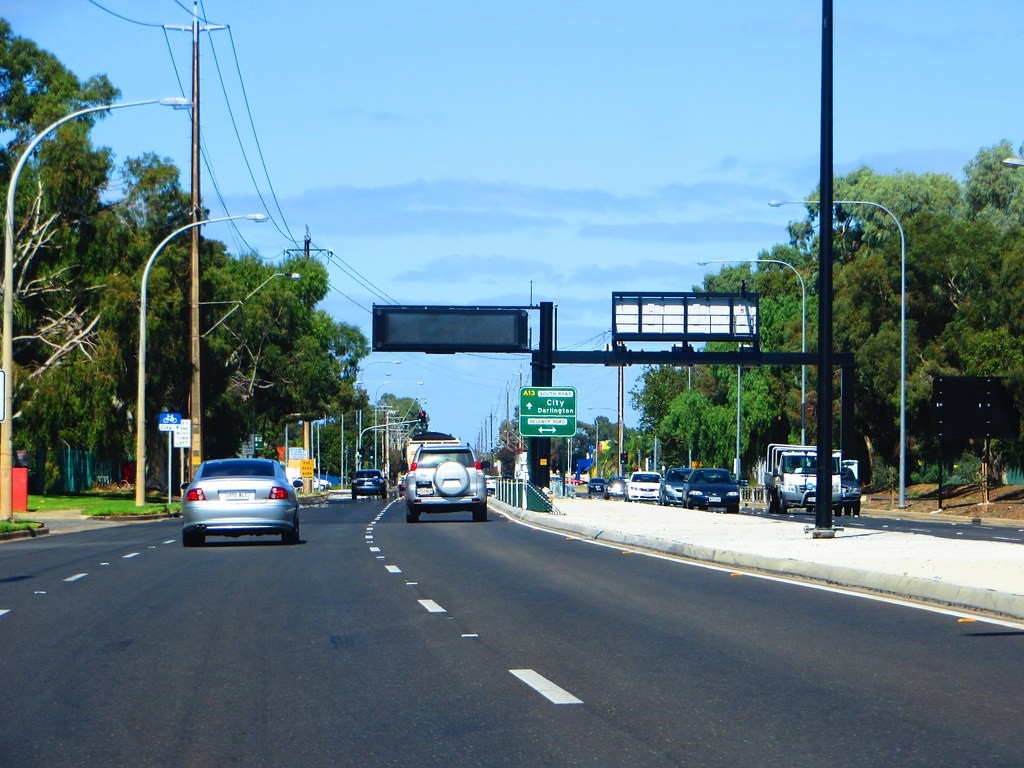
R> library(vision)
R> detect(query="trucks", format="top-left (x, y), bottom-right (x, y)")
top-left (764, 443), bottom-right (843, 514)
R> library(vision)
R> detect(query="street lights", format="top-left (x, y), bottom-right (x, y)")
top-left (190, 270), bottom-right (302, 479)
top-left (697, 259), bottom-right (806, 444)
top-left (135, 214), bottom-right (269, 507)
top-left (0, 98), bottom-right (193, 518)
top-left (587, 406), bottom-right (623, 476)
top-left (769, 199), bottom-right (906, 510)
top-left (357, 359), bottom-right (424, 491)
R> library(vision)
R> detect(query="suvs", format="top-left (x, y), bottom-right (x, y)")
top-left (834, 467), bottom-right (863, 516)
top-left (351, 468), bottom-right (389, 500)
top-left (405, 443), bottom-right (491, 522)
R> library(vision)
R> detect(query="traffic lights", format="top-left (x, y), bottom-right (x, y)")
top-left (620, 452), bottom-right (627, 466)
top-left (419, 410), bottom-right (426, 423)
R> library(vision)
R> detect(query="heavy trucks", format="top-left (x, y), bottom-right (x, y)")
top-left (405, 432), bottom-right (460, 471)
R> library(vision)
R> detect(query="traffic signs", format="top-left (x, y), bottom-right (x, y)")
top-left (518, 386), bottom-right (577, 438)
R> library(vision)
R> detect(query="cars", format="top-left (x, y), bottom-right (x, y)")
top-left (180, 458), bottom-right (304, 547)
top-left (398, 476), bottom-right (405, 490)
top-left (485, 474), bottom-right (498, 489)
top-left (682, 468), bottom-right (742, 514)
top-left (587, 471), bottom-right (663, 502)
top-left (313, 475), bottom-right (332, 491)
top-left (550, 476), bottom-right (583, 486)
top-left (658, 468), bottom-right (693, 506)
